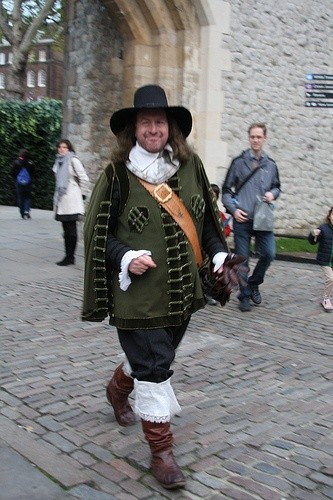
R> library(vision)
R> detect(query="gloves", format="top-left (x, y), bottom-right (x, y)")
top-left (223, 252), bottom-right (250, 290)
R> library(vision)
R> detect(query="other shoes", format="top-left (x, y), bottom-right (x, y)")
top-left (57, 255), bottom-right (75, 266)
top-left (208, 298), bottom-right (217, 305)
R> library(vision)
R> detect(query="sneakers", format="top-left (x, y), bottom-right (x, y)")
top-left (321, 299), bottom-right (332, 310)
top-left (248, 277), bottom-right (261, 304)
top-left (239, 296), bottom-right (252, 311)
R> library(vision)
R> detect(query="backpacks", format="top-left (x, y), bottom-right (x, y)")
top-left (17, 163), bottom-right (31, 186)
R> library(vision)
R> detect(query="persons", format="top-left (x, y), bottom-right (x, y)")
top-left (9, 149), bottom-right (37, 220)
top-left (52, 139), bottom-right (90, 267)
top-left (309, 207), bottom-right (332, 315)
top-left (209, 185), bottom-right (231, 239)
top-left (221, 121), bottom-right (281, 313)
top-left (81, 83), bottom-right (237, 490)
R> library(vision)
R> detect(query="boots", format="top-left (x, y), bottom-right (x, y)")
top-left (106, 363), bottom-right (137, 426)
top-left (143, 418), bottom-right (186, 488)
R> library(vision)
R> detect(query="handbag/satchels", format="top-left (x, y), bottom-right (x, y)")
top-left (199, 259), bottom-right (234, 308)
top-left (225, 194), bottom-right (239, 214)
top-left (252, 197), bottom-right (276, 232)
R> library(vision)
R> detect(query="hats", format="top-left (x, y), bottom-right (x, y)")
top-left (110, 85), bottom-right (193, 140)
